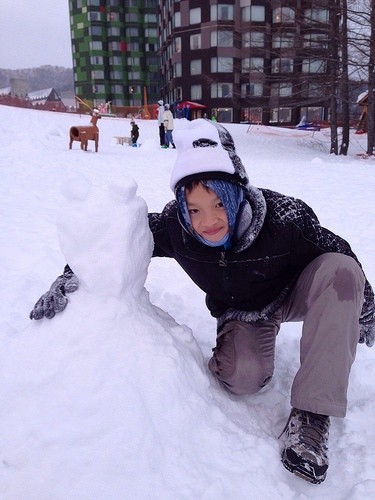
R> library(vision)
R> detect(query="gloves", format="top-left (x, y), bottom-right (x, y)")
top-left (29, 264), bottom-right (78, 320)
top-left (358, 321), bottom-right (375, 347)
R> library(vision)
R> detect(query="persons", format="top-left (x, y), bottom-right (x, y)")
top-left (28, 119), bottom-right (375, 486)
top-left (163, 103), bottom-right (176, 148)
top-left (130, 121), bottom-right (139, 147)
top-left (157, 100), bottom-right (165, 146)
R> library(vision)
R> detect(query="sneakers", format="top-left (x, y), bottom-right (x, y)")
top-left (277, 407), bottom-right (331, 484)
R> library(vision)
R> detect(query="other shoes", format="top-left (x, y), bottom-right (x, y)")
top-left (132, 143), bottom-right (137, 147)
top-left (172, 143), bottom-right (176, 148)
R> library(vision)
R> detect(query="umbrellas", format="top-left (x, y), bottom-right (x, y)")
top-left (174, 100), bottom-right (207, 121)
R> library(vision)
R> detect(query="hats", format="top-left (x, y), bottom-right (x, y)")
top-left (130, 121), bottom-right (134, 124)
top-left (165, 103), bottom-right (169, 110)
top-left (158, 100), bottom-right (163, 106)
top-left (170, 118), bottom-right (249, 202)
